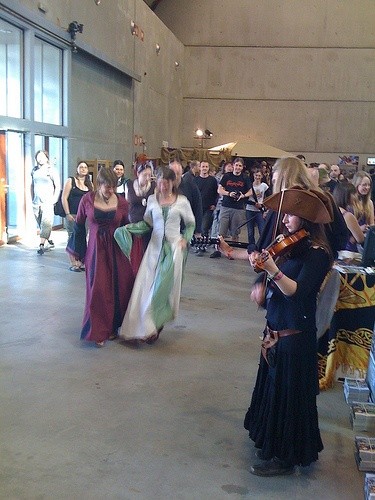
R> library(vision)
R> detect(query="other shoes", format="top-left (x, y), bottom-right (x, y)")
top-left (194, 247), bottom-right (205, 257)
top-left (210, 251), bottom-right (221, 258)
top-left (80, 265), bottom-right (86, 271)
top-left (47, 238), bottom-right (55, 245)
top-left (249, 458), bottom-right (294, 477)
top-left (319, 375), bottom-right (336, 391)
top-left (255, 448), bottom-right (276, 459)
top-left (109, 328), bottom-right (119, 340)
top-left (38, 244), bottom-right (45, 254)
top-left (146, 327), bottom-right (163, 344)
top-left (229, 256), bottom-right (234, 260)
top-left (70, 265), bottom-right (81, 272)
top-left (95, 341), bottom-right (104, 346)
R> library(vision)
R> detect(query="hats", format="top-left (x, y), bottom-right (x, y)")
top-left (262, 185), bottom-right (334, 225)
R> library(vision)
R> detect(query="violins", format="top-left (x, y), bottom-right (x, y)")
top-left (255, 227), bottom-right (308, 273)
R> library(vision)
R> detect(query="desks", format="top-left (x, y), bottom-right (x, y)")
top-left (318, 262), bottom-right (375, 391)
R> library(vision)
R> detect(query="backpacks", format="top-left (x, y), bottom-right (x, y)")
top-left (54, 177), bottom-right (76, 218)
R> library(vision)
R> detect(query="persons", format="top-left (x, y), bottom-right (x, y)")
top-left (216, 157), bottom-right (344, 356)
top-left (112, 159), bottom-right (132, 200)
top-left (113, 168), bottom-right (195, 344)
top-left (127, 154), bottom-right (157, 248)
top-left (244, 185), bottom-right (339, 476)
top-left (61, 160), bottom-right (94, 272)
top-left (66, 167), bottom-right (135, 348)
top-left (166, 155), bottom-right (375, 260)
top-left (29, 150), bottom-right (60, 255)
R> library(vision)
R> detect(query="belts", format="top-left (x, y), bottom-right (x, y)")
top-left (260, 328), bottom-right (302, 364)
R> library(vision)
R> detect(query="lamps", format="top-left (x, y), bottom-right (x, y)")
top-left (206, 130), bottom-right (212, 137)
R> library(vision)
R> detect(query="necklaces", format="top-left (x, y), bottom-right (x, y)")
top-left (102, 192), bottom-right (110, 205)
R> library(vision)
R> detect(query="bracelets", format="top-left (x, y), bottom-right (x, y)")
top-left (267, 269), bottom-right (283, 282)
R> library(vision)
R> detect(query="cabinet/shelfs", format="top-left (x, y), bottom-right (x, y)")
top-left (86, 159), bottom-right (109, 189)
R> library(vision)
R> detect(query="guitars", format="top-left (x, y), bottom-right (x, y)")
top-left (189, 235), bottom-right (256, 250)
